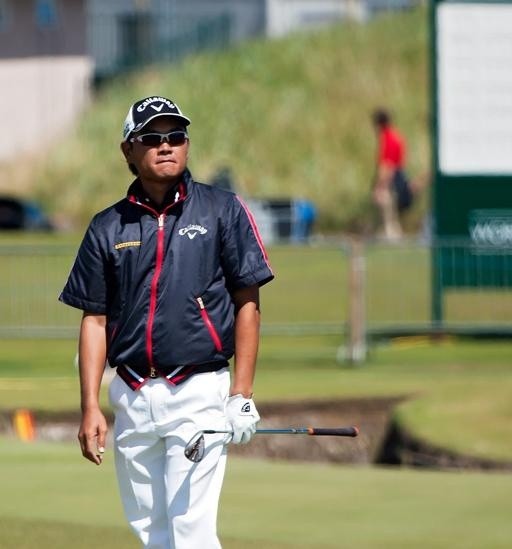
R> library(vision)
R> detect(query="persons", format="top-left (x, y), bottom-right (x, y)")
top-left (56, 95), bottom-right (277, 549)
top-left (369, 106), bottom-right (407, 241)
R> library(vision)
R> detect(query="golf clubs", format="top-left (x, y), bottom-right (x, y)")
top-left (185, 428), bottom-right (359, 462)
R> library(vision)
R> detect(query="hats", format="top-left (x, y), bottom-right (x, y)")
top-left (123, 96), bottom-right (190, 141)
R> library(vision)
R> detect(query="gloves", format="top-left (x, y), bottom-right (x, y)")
top-left (224, 394), bottom-right (260, 445)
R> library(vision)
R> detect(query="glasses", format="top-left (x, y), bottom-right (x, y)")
top-left (130, 131), bottom-right (190, 146)
